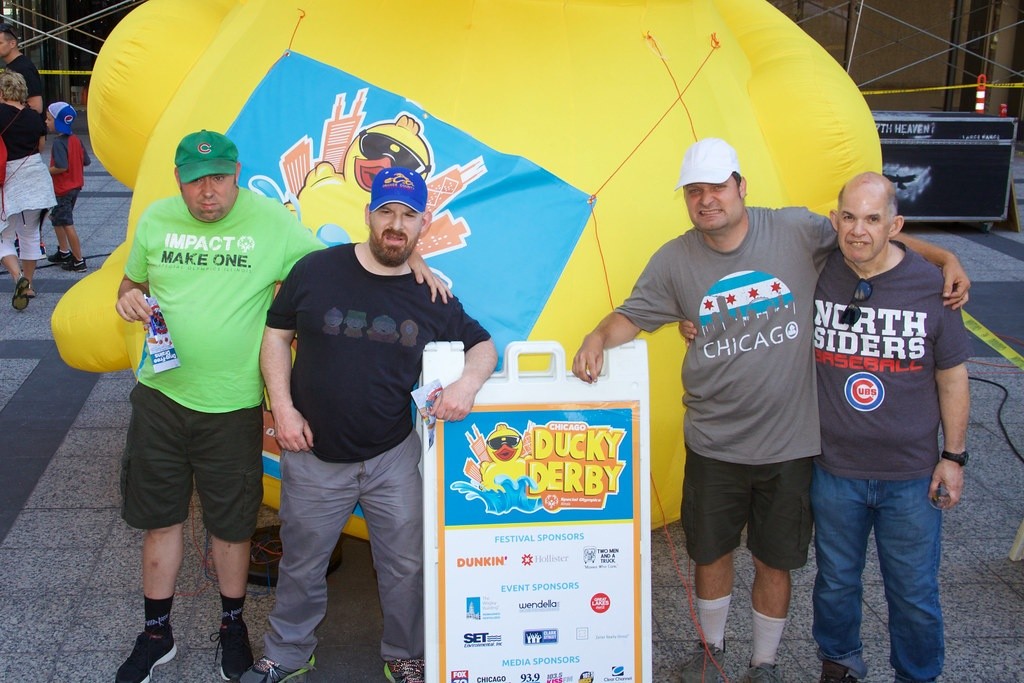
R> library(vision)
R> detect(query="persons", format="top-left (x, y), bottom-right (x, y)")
top-left (572, 137), bottom-right (971, 683)
top-left (238, 166), bottom-right (498, 682)
top-left (116, 129), bottom-right (453, 683)
top-left (0, 22), bottom-right (91, 309)
top-left (679, 172), bottom-right (970, 683)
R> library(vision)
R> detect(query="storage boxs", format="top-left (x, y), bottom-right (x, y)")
top-left (870, 110), bottom-right (1020, 233)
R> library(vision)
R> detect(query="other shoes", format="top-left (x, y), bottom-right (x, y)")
top-left (817, 660), bottom-right (857, 683)
top-left (11, 277), bottom-right (30, 310)
top-left (61, 256), bottom-right (87, 272)
top-left (47, 246), bottom-right (73, 262)
top-left (26, 283), bottom-right (37, 297)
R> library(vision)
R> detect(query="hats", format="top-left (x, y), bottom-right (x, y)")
top-left (368, 167), bottom-right (428, 213)
top-left (174, 130), bottom-right (239, 184)
top-left (49, 102), bottom-right (77, 134)
top-left (673, 138), bottom-right (741, 192)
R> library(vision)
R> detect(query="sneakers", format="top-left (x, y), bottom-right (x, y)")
top-left (209, 615), bottom-right (256, 681)
top-left (115, 625), bottom-right (177, 683)
top-left (239, 652), bottom-right (315, 683)
top-left (745, 655), bottom-right (780, 683)
top-left (384, 658), bottom-right (425, 683)
top-left (679, 638), bottom-right (726, 683)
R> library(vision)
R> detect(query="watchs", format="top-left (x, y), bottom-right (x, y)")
top-left (941, 450), bottom-right (969, 466)
